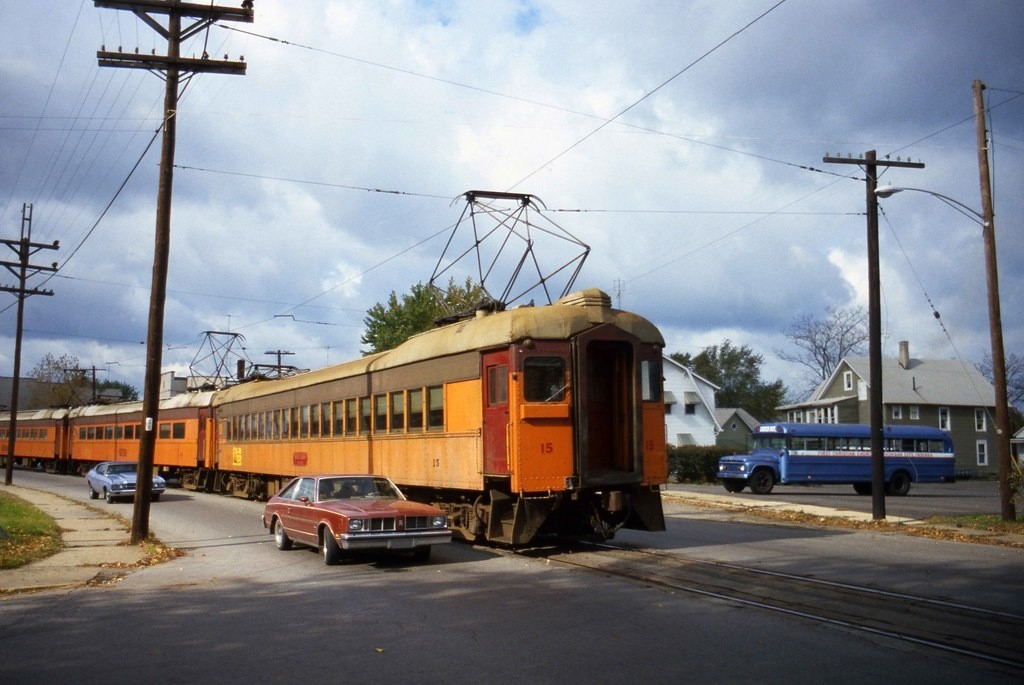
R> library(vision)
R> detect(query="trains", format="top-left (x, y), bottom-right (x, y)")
top-left (0, 284), bottom-right (668, 550)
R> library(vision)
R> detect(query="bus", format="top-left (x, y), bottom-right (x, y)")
top-left (717, 422), bottom-right (955, 495)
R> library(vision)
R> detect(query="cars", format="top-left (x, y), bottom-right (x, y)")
top-left (84, 460), bottom-right (166, 503)
top-left (262, 475), bottom-right (453, 566)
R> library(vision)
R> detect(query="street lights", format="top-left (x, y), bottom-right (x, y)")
top-left (874, 179), bottom-right (1014, 530)
